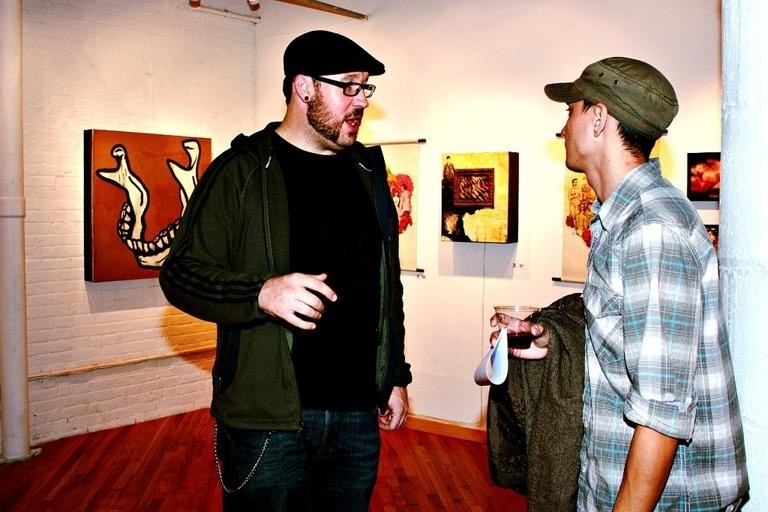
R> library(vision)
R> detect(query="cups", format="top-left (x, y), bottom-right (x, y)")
top-left (494, 304), bottom-right (543, 349)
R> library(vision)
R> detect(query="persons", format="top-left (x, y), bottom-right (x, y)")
top-left (161, 30), bottom-right (410, 512)
top-left (393, 184), bottom-right (412, 226)
top-left (566, 175), bottom-right (594, 237)
top-left (489, 55), bottom-right (747, 512)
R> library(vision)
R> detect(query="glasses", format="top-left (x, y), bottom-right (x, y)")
top-left (312, 77), bottom-right (377, 97)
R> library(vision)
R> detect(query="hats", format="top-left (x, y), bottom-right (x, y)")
top-left (543, 57), bottom-right (679, 139)
top-left (282, 31), bottom-right (386, 77)
